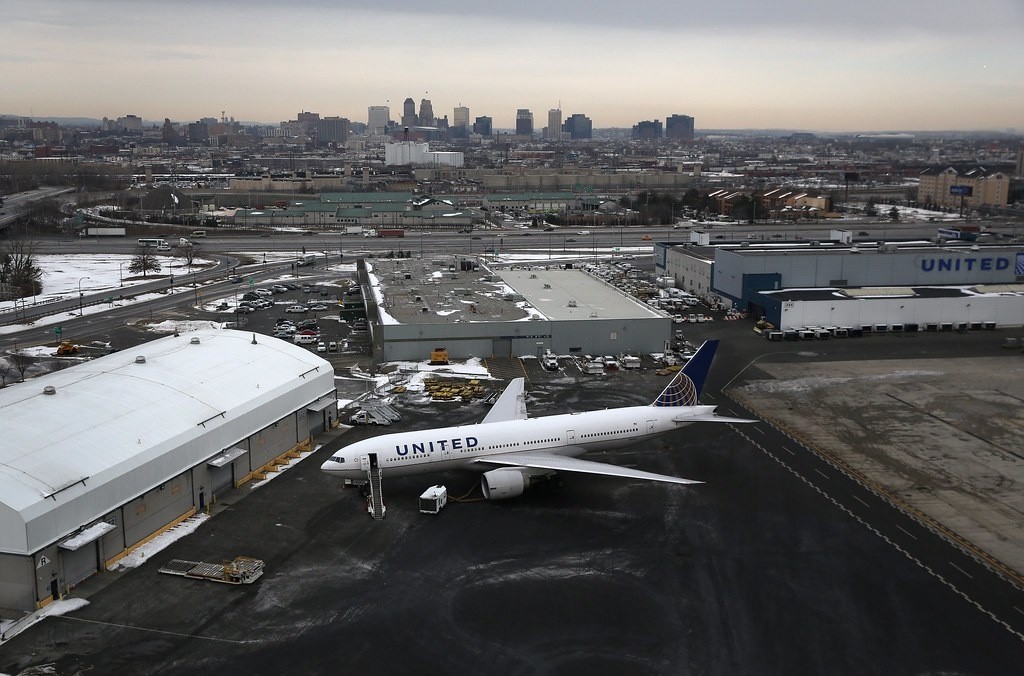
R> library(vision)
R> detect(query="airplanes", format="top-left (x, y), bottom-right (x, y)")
top-left (321, 338), bottom-right (761, 502)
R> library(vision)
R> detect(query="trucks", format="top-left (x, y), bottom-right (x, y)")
top-left (172, 238), bottom-right (193, 249)
top-left (296, 254), bottom-right (316, 268)
top-left (340, 226), bottom-right (362, 235)
top-left (578, 230), bottom-right (590, 235)
top-left (674, 221), bottom-right (693, 229)
top-left (190, 231), bottom-right (206, 238)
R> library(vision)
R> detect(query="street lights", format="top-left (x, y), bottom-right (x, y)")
top-left (191, 268), bottom-right (196, 282)
top-left (78, 276), bottom-right (90, 293)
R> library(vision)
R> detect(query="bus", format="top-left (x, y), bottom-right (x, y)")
top-left (138, 239), bottom-right (168, 248)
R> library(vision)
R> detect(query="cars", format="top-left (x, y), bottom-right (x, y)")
top-left (302, 230), bottom-right (313, 236)
top-left (472, 236), bottom-right (482, 240)
top-left (543, 227), bottom-right (554, 231)
top-left (497, 233), bottom-right (508, 237)
top-left (565, 238), bottom-right (577, 242)
top-left (235, 282), bottom-right (338, 354)
top-left (642, 224), bottom-right (870, 245)
top-left (520, 233), bottom-right (532, 237)
top-left (458, 228), bottom-right (473, 233)
top-left (423, 231), bottom-right (432, 236)
top-left (484, 247), bottom-right (496, 253)
top-left (260, 233), bottom-right (270, 237)
top-left (157, 246), bottom-right (170, 251)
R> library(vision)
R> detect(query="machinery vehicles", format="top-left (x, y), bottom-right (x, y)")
top-left (56, 341), bottom-right (80, 355)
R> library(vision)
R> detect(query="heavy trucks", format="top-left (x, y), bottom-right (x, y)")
top-left (364, 229), bottom-right (404, 238)
